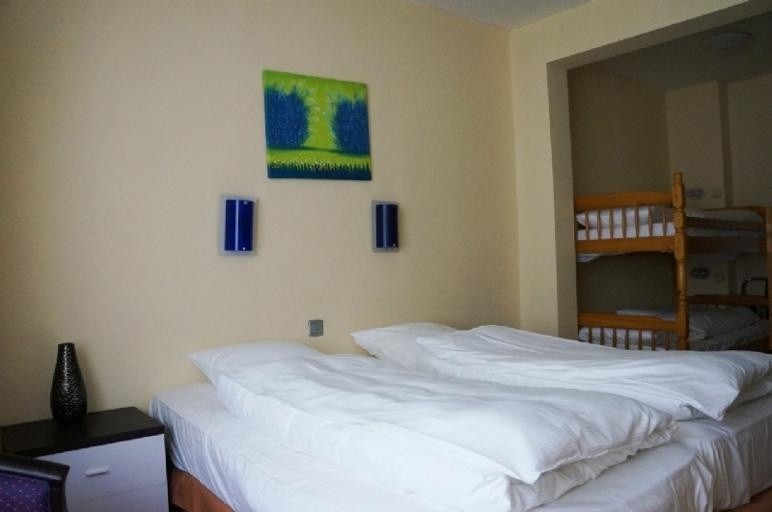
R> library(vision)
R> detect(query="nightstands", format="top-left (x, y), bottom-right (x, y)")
top-left (0, 405), bottom-right (172, 512)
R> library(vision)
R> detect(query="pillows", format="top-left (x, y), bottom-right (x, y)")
top-left (576, 204), bottom-right (669, 228)
top-left (348, 319), bottom-right (459, 361)
top-left (182, 338), bottom-right (328, 392)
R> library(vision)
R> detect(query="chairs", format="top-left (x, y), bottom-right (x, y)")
top-left (1, 450), bottom-right (71, 512)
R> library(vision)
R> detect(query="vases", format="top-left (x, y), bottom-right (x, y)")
top-left (47, 341), bottom-right (90, 426)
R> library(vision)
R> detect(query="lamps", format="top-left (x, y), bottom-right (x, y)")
top-left (218, 194), bottom-right (257, 257)
top-left (370, 199), bottom-right (400, 253)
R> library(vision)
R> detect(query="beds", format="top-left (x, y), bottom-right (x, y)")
top-left (349, 317), bottom-right (772, 512)
top-left (574, 171), bottom-right (772, 353)
top-left (141, 333), bottom-right (717, 512)
